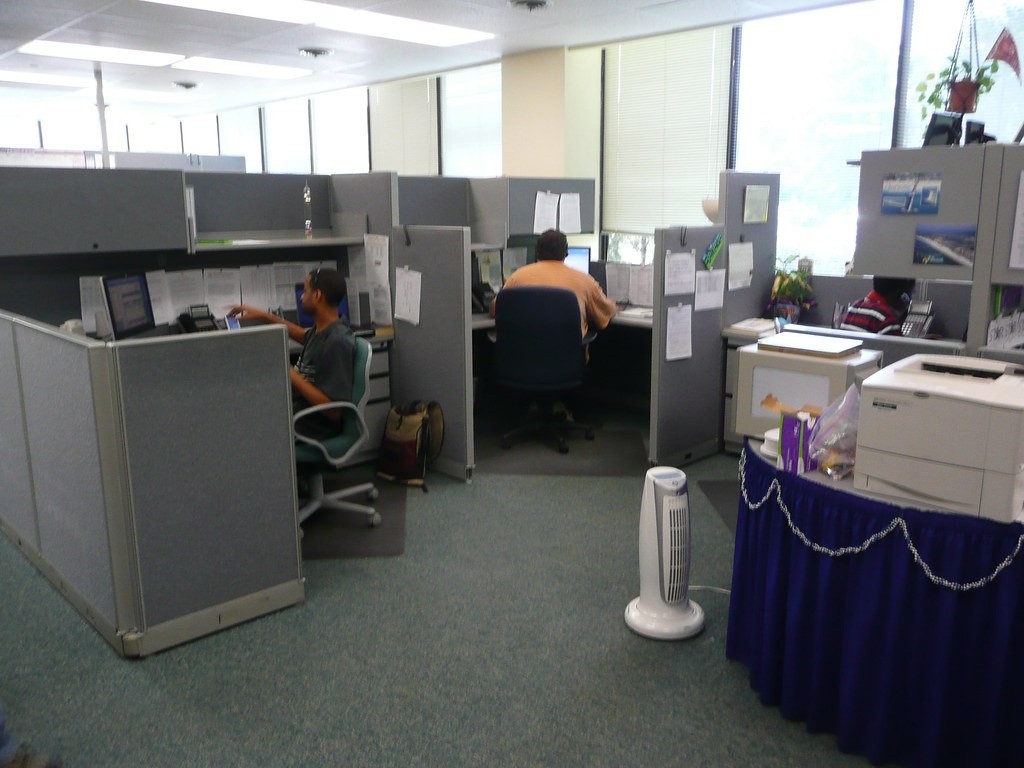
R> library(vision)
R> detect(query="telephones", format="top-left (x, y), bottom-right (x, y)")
top-left (177, 302), bottom-right (222, 333)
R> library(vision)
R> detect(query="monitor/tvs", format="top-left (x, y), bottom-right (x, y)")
top-left (295, 282), bottom-right (350, 328)
top-left (535, 246), bottom-right (591, 275)
top-left (100, 270), bottom-right (155, 341)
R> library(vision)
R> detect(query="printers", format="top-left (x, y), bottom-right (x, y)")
top-left (853, 352), bottom-right (1024, 524)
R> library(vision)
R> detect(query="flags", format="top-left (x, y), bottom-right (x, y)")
top-left (985, 27), bottom-right (1023, 86)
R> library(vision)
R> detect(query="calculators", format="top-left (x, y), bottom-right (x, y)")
top-left (900, 299), bottom-right (937, 338)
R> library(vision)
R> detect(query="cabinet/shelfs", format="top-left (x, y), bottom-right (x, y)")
top-left (339, 338), bottom-right (392, 465)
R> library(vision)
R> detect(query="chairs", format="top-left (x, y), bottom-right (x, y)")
top-left (487, 289), bottom-right (600, 454)
top-left (289, 337), bottom-right (383, 541)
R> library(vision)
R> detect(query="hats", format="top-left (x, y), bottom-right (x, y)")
top-left (536, 229), bottom-right (568, 257)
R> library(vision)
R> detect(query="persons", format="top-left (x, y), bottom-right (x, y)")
top-left (227, 267), bottom-right (357, 445)
top-left (489, 229), bottom-right (616, 367)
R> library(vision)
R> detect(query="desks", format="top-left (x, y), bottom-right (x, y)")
top-left (471, 305), bottom-right (653, 331)
top-left (724, 437), bottom-right (1024, 768)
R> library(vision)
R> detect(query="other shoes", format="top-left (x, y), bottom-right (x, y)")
top-left (525, 401), bottom-right (541, 423)
top-left (552, 400), bottom-right (574, 423)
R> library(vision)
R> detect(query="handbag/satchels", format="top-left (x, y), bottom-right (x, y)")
top-left (375, 400), bottom-right (445, 487)
top-left (809, 382), bottom-right (860, 482)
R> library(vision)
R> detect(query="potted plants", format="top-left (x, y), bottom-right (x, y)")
top-left (767, 253), bottom-right (815, 325)
top-left (916, 55), bottom-right (1000, 139)
